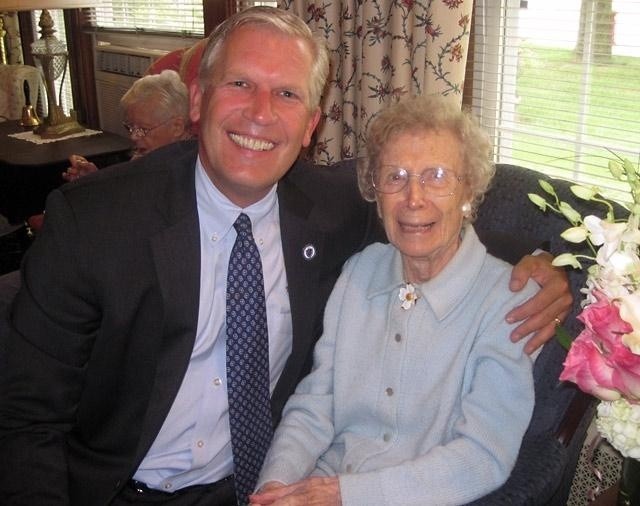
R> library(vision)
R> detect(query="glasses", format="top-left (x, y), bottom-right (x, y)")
top-left (122, 120), bottom-right (167, 138)
top-left (370, 161), bottom-right (467, 195)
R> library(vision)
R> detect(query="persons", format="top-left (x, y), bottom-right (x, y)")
top-left (244, 90), bottom-right (548, 506)
top-left (0, 68), bottom-right (198, 316)
top-left (0, 3), bottom-right (575, 504)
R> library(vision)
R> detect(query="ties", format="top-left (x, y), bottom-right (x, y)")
top-left (225, 214), bottom-right (273, 504)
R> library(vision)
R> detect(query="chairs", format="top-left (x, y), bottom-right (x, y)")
top-left (1, 65), bottom-right (47, 120)
top-left (330, 157), bottom-right (630, 506)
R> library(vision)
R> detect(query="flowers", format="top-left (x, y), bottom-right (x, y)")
top-left (528, 147), bottom-right (639, 466)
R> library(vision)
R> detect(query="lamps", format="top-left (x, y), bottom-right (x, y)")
top-left (0, 0), bottom-right (90, 139)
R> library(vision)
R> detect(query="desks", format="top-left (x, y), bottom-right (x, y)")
top-left (0, 121), bottom-right (129, 166)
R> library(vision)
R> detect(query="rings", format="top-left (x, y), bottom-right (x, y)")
top-left (553, 315), bottom-right (563, 325)
